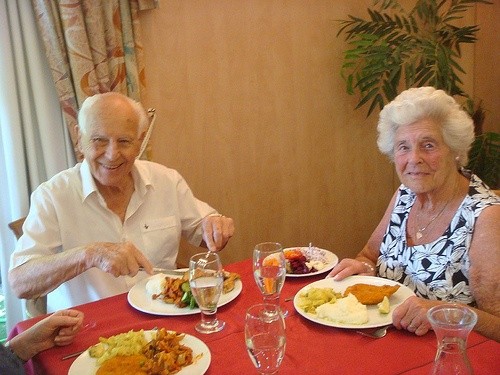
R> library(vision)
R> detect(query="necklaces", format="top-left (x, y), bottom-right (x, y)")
top-left (415, 198), bottom-right (449, 240)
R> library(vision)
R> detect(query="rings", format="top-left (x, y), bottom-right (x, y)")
top-left (410, 322), bottom-right (418, 329)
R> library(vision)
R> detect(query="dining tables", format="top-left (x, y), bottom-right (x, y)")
top-left (5, 257), bottom-right (500, 375)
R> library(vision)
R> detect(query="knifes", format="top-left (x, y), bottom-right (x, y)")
top-left (138, 266), bottom-right (188, 276)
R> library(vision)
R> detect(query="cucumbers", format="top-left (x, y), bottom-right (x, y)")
top-left (180, 282), bottom-right (198, 308)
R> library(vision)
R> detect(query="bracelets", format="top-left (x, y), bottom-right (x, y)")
top-left (5, 340), bottom-right (28, 364)
top-left (361, 261), bottom-right (374, 272)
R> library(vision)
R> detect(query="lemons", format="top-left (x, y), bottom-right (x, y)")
top-left (379, 296), bottom-right (390, 314)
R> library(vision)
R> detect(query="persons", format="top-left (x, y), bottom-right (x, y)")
top-left (0, 310), bottom-right (85, 374)
top-left (330, 86), bottom-right (500, 342)
top-left (7, 93), bottom-right (235, 315)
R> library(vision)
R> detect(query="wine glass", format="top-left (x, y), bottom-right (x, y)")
top-left (253, 242), bottom-right (287, 321)
top-left (243, 303), bottom-right (288, 375)
top-left (188, 253), bottom-right (226, 334)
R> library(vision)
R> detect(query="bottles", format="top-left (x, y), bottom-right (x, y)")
top-left (426, 304), bottom-right (477, 375)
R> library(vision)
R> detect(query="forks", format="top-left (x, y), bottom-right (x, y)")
top-left (195, 250), bottom-right (213, 270)
top-left (358, 326), bottom-right (396, 338)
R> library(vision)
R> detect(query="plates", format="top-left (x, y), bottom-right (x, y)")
top-left (127, 268), bottom-right (243, 315)
top-left (68, 330), bottom-right (211, 375)
top-left (293, 276), bottom-right (418, 328)
top-left (263, 246), bottom-right (339, 277)
